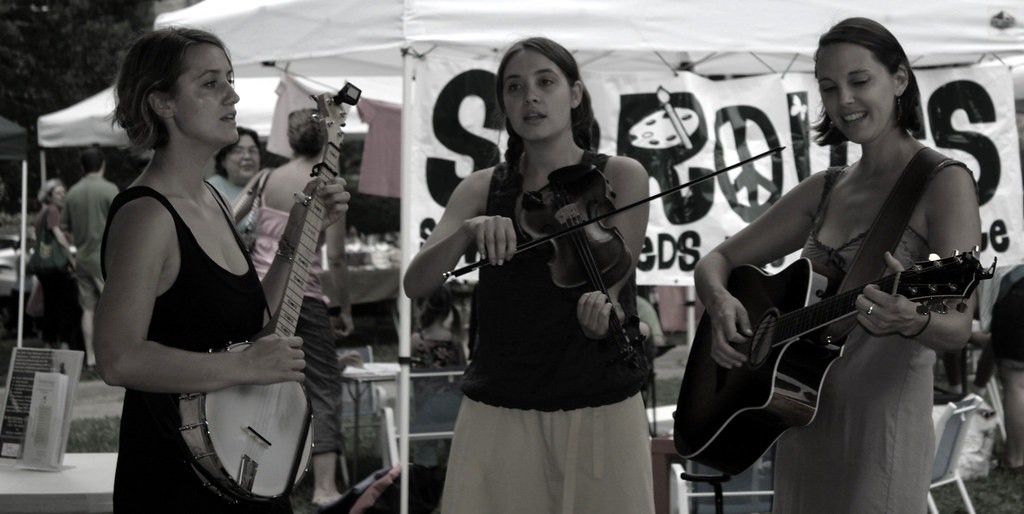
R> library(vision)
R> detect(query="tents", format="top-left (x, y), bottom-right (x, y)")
top-left (38, 75), bottom-right (402, 216)
top-left (155, 0), bottom-right (1024, 514)
top-left (0, 115), bottom-right (30, 349)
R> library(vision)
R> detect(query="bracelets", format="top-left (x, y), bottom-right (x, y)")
top-left (275, 234), bottom-right (294, 262)
top-left (901, 310), bottom-right (931, 338)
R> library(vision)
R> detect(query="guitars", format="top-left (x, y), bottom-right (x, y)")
top-left (672, 246), bottom-right (1001, 478)
top-left (175, 81), bottom-right (362, 507)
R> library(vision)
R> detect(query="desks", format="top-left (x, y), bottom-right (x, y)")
top-left (342, 362), bottom-right (401, 485)
top-left (319, 268), bottom-right (398, 334)
top-left (0, 453), bottom-right (118, 514)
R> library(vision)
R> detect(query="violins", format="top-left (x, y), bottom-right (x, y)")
top-left (517, 164), bottom-right (652, 378)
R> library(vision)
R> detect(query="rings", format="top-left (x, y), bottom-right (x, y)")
top-left (867, 304), bottom-right (874, 315)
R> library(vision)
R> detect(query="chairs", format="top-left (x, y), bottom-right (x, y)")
top-left (927, 393), bottom-right (982, 514)
top-left (669, 444), bottom-right (776, 514)
top-left (381, 363), bottom-right (465, 469)
top-left (337, 345), bottom-right (383, 468)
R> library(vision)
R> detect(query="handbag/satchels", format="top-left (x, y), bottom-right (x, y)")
top-left (232, 167), bottom-right (274, 253)
top-left (24, 206), bottom-right (71, 276)
top-left (931, 392), bottom-right (984, 483)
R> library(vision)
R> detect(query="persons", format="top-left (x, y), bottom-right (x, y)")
top-left (33, 147), bottom-right (120, 367)
top-left (693, 16), bottom-right (982, 514)
top-left (403, 37), bottom-right (657, 514)
top-left (942, 266), bottom-right (1024, 471)
top-left (92, 27), bottom-right (351, 514)
top-left (234, 107), bottom-right (354, 506)
top-left (205, 126), bottom-right (262, 204)
